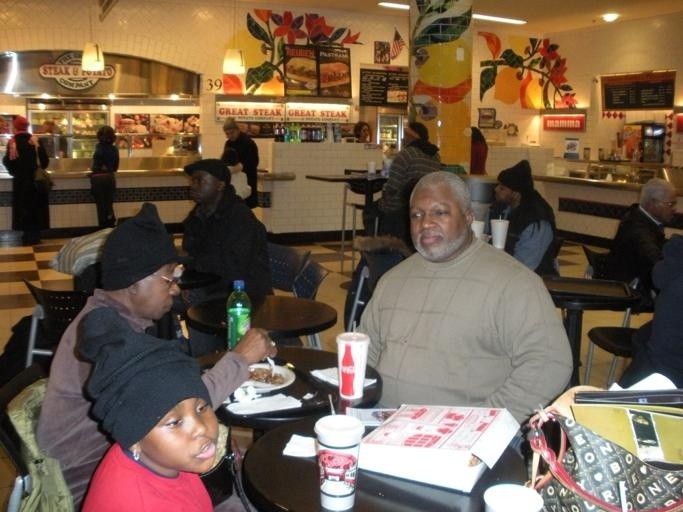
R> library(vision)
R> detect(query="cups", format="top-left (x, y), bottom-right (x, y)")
top-left (383, 159), bottom-right (392, 177)
top-left (336, 332), bottom-right (370, 400)
top-left (490, 219), bottom-right (509, 249)
top-left (368, 159), bottom-right (376, 175)
top-left (483, 485), bottom-right (543, 512)
top-left (471, 222), bottom-right (484, 238)
top-left (314, 415), bottom-right (366, 512)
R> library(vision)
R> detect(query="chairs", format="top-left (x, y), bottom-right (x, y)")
top-left (585, 295), bottom-right (641, 390)
top-left (555, 237), bottom-right (564, 275)
top-left (341, 169), bottom-right (390, 276)
top-left (583, 244), bottom-right (659, 374)
top-left (23, 277), bottom-right (89, 369)
top-left (265, 241), bottom-right (311, 292)
top-left (0, 363), bottom-right (50, 477)
top-left (292, 258), bottom-right (334, 350)
top-left (404, 179), bottom-right (420, 195)
top-left (9, 475), bottom-right (73, 512)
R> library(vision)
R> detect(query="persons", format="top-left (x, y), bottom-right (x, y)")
top-left (484, 160), bottom-right (561, 277)
top-left (221, 147), bottom-right (252, 200)
top-left (582, 178), bottom-right (677, 310)
top-left (353, 121), bottom-right (369, 143)
top-left (173, 159), bottom-right (271, 306)
top-left (612, 234), bottom-right (683, 389)
top-left (3, 115), bottom-right (55, 247)
top-left (471, 127), bottom-right (488, 175)
top-left (73, 306), bottom-right (219, 512)
top-left (355, 172), bottom-right (574, 424)
top-left (33, 203), bottom-right (278, 512)
top-left (374, 122), bottom-right (441, 234)
top-left (89, 126), bottom-right (120, 228)
top-left (221, 117), bottom-right (259, 209)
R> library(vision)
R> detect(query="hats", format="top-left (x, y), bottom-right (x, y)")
top-left (74, 303), bottom-right (212, 452)
top-left (222, 117), bottom-right (238, 132)
top-left (407, 120), bottom-right (428, 139)
top-left (183, 159), bottom-right (231, 184)
top-left (97, 126), bottom-right (116, 144)
top-left (99, 201), bottom-right (185, 291)
top-left (13, 116), bottom-right (30, 131)
top-left (497, 159), bottom-right (535, 195)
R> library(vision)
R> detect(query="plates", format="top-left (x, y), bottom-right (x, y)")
top-left (243, 364), bottom-right (296, 393)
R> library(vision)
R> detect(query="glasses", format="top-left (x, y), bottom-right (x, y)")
top-left (650, 198), bottom-right (676, 211)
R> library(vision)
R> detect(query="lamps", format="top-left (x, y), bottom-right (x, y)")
top-left (222, 1), bottom-right (245, 78)
top-left (81, 0), bottom-right (106, 75)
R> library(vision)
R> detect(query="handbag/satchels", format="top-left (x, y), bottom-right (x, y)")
top-left (531, 379), bottom-right (682, 512)
top-left (34, 139), bottom-right (55, 198)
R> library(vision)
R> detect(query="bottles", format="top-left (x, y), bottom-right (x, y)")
top-left (273, 123), bottom-right (327, 143)
top-left (228, 280), bottom-right (250, 352)
top-left (334, 122), bottom-right (342, 143)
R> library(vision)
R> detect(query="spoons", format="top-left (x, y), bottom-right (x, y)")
top-left (266, 356), bottom-right (276, 378)
top-left (266, 355), bottom-right (275, 378)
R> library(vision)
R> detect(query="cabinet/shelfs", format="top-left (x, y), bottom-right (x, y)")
top-left (563, 158), bottom-right (660, 186)
